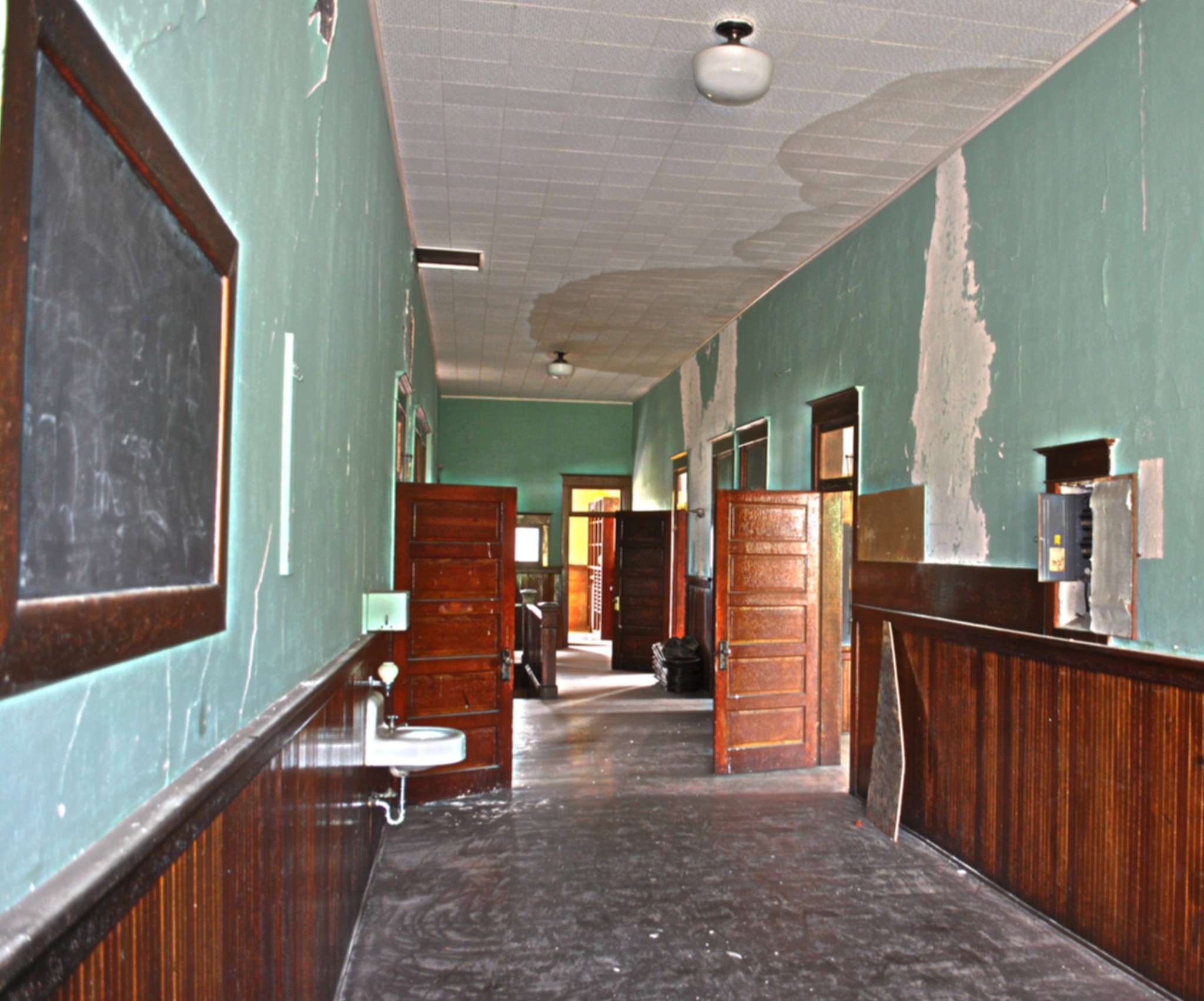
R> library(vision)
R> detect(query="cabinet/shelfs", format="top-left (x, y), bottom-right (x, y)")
top-left (587, 497), bottom-right (620, 640)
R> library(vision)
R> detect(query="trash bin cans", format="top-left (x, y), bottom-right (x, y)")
top-left (662, 657), bottom-right (706, 693)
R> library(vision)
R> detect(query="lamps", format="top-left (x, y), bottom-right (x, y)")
top-left (692, 18), bottom-right (773, 108)
top-left (546, 350), bottom-right (574, 380)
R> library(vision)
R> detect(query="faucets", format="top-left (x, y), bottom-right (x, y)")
top-left (367, 661), bottom-right (400, 697)
top-left (386, 714), bottom-right (409, 733)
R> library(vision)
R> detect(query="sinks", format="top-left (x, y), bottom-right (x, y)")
top-left (364, 725), bottom-right (467, 768)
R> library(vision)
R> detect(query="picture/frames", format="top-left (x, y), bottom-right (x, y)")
top-left (0, 0), bottom-right (242, 696)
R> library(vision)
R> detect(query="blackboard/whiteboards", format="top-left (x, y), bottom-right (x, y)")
top-left (0, 0), bottom-right (239, 699)
